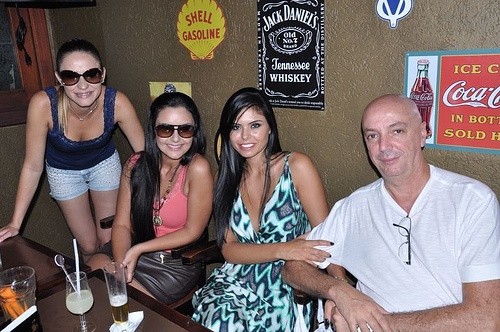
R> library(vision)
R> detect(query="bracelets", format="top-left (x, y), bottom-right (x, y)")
top-left (334, 275), bottom-right (348, 282)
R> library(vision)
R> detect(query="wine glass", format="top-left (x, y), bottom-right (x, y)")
top-left (66, 271), bottom-right (96, 332)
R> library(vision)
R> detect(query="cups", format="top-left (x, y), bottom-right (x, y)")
top-left (0, 266), bottom-right (37, 322)
top-left (102, 262), bottom-right (129, 324)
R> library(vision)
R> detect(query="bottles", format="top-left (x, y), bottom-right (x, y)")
top-left (409, 60), bottom-right (434, 139)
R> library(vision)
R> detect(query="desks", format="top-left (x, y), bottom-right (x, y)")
top-left (0, 234), bottom-right (215, 332)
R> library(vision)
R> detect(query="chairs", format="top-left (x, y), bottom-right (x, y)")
top-left (93, 214), bottom-right (209, 309)
top-left (180, 239), bottom-right (327, 332)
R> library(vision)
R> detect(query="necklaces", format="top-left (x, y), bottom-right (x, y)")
top-left (67, 100), bottom-right (98, 122)
top-left (152, 163), bottom-right (181, 225)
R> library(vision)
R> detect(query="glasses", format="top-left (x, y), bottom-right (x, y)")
top-left (153, 122), bottom-right (197, 138)
top-left (57, 68), bottom-right (105, 86)
top-left (392, 216), bottom-right (411, 265)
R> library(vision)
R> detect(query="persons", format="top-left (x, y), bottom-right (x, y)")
top-left (87, 91), bottom-right (214, 306)
top-left (281, 94), bottom-right (500, 332)
top-left (0, 38), bottom-right (146, 265)
top-left (189, 86), bottom-right (349, 332)
